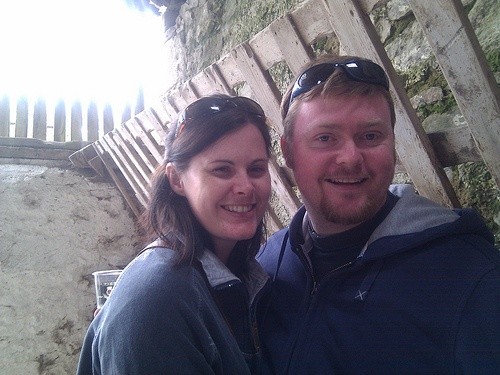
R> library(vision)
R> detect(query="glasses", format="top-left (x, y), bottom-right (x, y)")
top-left (175, 95), bottom-right (264, 142)
top-left (285, 59), bottom-right (390, 114)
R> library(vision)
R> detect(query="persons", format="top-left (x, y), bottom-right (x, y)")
top-left (76, 94), bottom-right (273, 375)
top-left (94, 56), bottom-right (500, 375)
